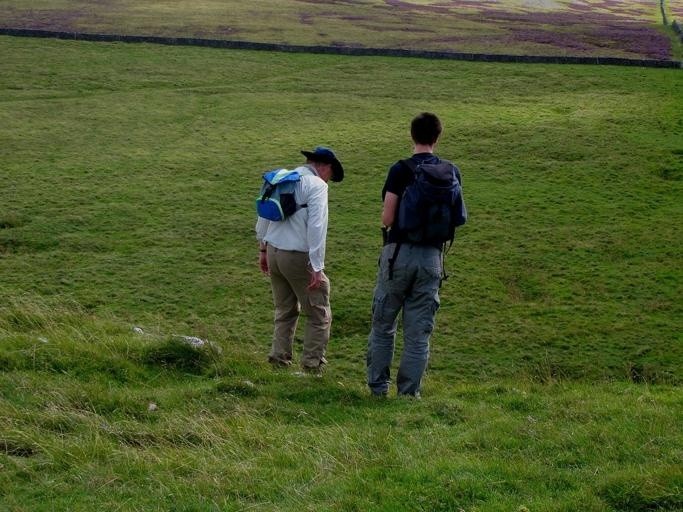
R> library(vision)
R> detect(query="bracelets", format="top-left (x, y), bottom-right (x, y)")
top-left (258, 247), bottom-right (266, 253)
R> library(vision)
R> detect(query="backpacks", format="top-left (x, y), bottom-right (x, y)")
top-left (254, 167), bottom-right (309, 221)
top-left (396, 158), bottom-right (460, 244)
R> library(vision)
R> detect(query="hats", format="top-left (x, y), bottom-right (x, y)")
top-left (298, 144), bottom-right (344, 184)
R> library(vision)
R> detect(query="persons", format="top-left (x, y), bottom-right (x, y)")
top-left (362, 111), bottom-right (463, 403)
top-left (255, 146), bottom-right (345, 377)
